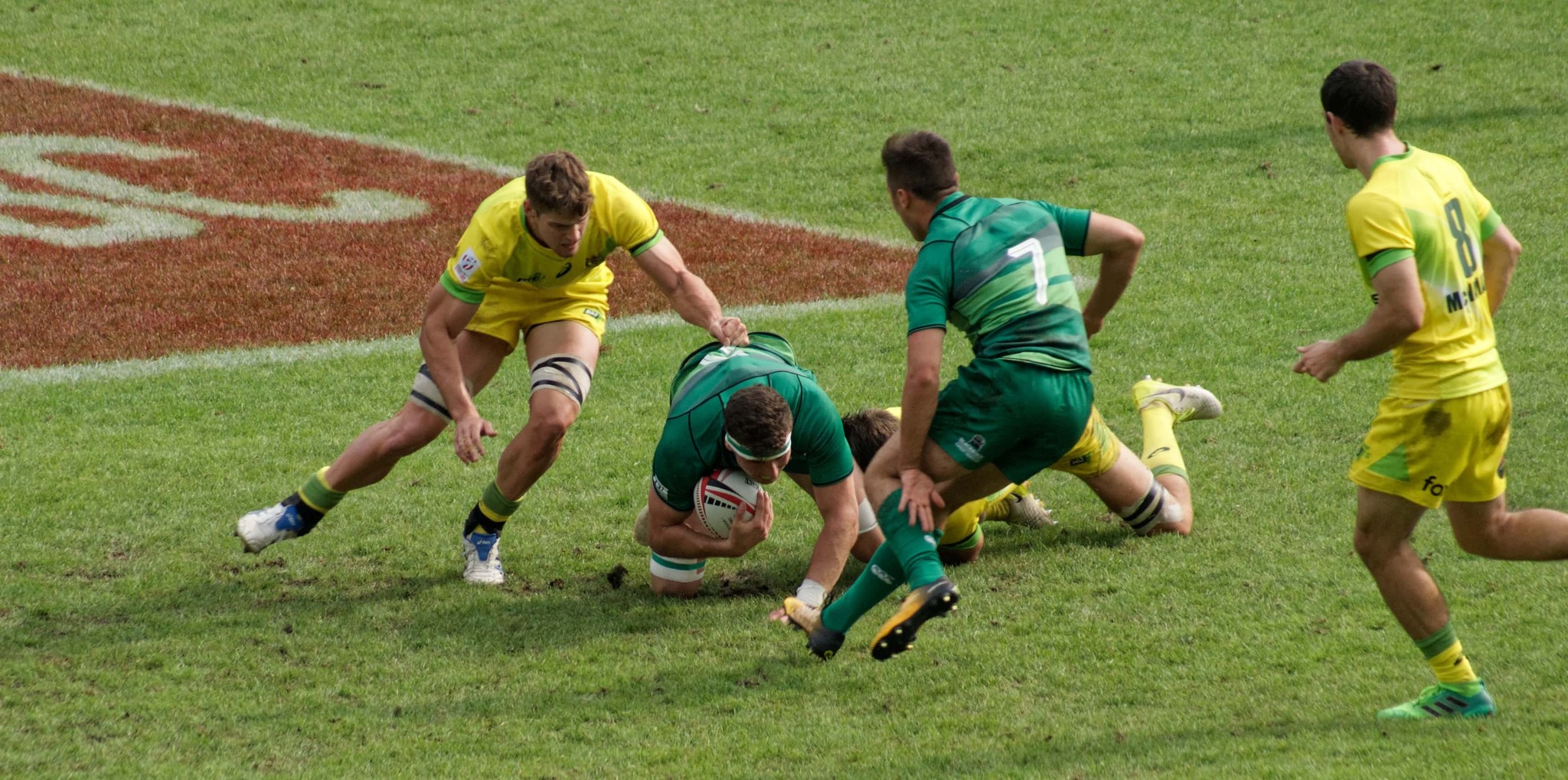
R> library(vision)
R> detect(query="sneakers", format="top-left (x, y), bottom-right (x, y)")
top-left (999, 481), bottom-right (1057, 528)
top-left (1132, 372), bottom-right (1224, 424)
top-left (1375, 677), bottom-right (1496, 718)
top-left (462, 531), bottom-right (505, 586)
top-left (784, 590), bottom-right (844, 664)
top-left (236, 499), bottom-right (310, 552)
top-left (871, 580), bottom-right (958, 664)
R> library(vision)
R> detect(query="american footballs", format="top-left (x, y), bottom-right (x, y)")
top-left (693, 468), bottom-right (775, 540)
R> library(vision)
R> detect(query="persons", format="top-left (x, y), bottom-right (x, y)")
top-left (843, 376), bottom-right (1224, 561)
top-left (235, 148), bottom-right (751, 587)
top-left (650, 330), bottom-right (888, 631)
top-left (1290, 61), bottom-right (1568, 722)
top-left (782, 131), bottom-right (1145, 658)
